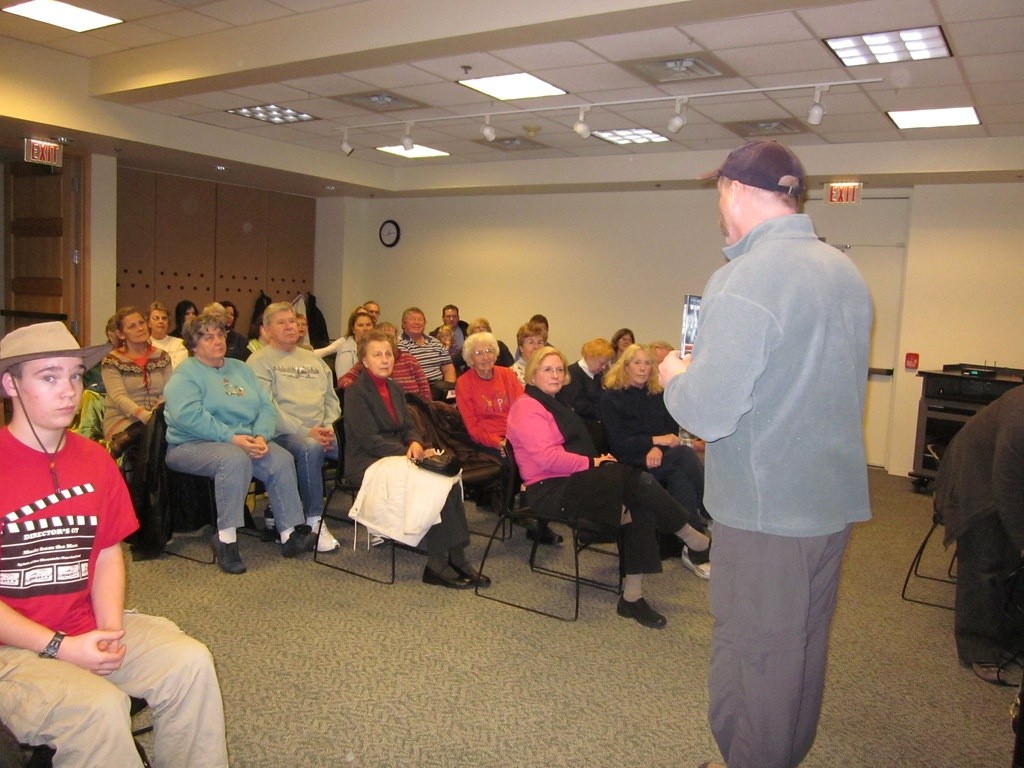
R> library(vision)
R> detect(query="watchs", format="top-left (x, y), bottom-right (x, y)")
top-left (39, 630), bottom-right (66, 659)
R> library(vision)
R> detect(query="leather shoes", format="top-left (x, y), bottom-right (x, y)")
top-left (422, 552), bottom-right (491, 589)
top-left (617, 599), bottom-right (667, 629)
top-left (688, 537), bottom-right (712, 565)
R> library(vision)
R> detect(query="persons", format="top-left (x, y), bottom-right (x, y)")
top-left (0, 320), bottom-right (230, 768)
top-left (655, 141), bottom-right (872, 768)
top-left (934, 384), bottom-right (1024, 683)
top-left (343, 329), bottom-right (492, 588)
top-left (506, 346), bottom-right (712, 629)
top-left (100, 300), bottom-right (706, 560)
top-left (163, 314), bottom-right (318, 574)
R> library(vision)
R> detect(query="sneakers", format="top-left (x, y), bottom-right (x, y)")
top-left (681, 544), bottom-right (711, 579)
top-left (306, 517), bottom-right (341, 554)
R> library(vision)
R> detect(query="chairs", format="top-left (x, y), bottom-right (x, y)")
top-left (0, 380), bottom-right (660, 768)
top-left (897, 437), bottom-right (958, 612)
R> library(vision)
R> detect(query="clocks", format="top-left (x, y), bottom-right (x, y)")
top-left (379, 219), bottom-right (401, 248)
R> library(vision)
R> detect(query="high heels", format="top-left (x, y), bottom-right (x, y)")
top-left (210, 532), bottom-right (246, 574)
top-left (280, 531), bottom-right (318, 558)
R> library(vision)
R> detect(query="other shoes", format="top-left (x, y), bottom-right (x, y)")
top-left (512, 492), bottom-right (531, 513)
top-left (526, 527), bottom-right (563, 544)
top-left (972, 662), bottom-right (1007, 684)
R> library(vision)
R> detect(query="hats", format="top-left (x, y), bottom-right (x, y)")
top-left (0, 321), bottom-right (113, 399)
top-left (697, 139), bottom-right (807, 194)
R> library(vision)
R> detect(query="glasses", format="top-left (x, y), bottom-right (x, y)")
top-left (152, 315), bottom-right (170, 321)
top-left (475, 347), bottom-right (495, 360)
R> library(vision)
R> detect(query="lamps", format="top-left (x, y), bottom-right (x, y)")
top-left (400, 123), bottom-right (414, 151)
top-left (807, 88), bottom-right (823, 128)
top-left (666, 101), bottom-right (688, 137)
top-left (574, 110), bottom-right (591, 140)
top-left (341, 131), bottom-right (354, 157)
top-left (479, 117), bottom-right (495, 142)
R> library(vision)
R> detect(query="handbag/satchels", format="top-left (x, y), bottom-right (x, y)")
top-left (410, 455), bottom-right (461, 477)
